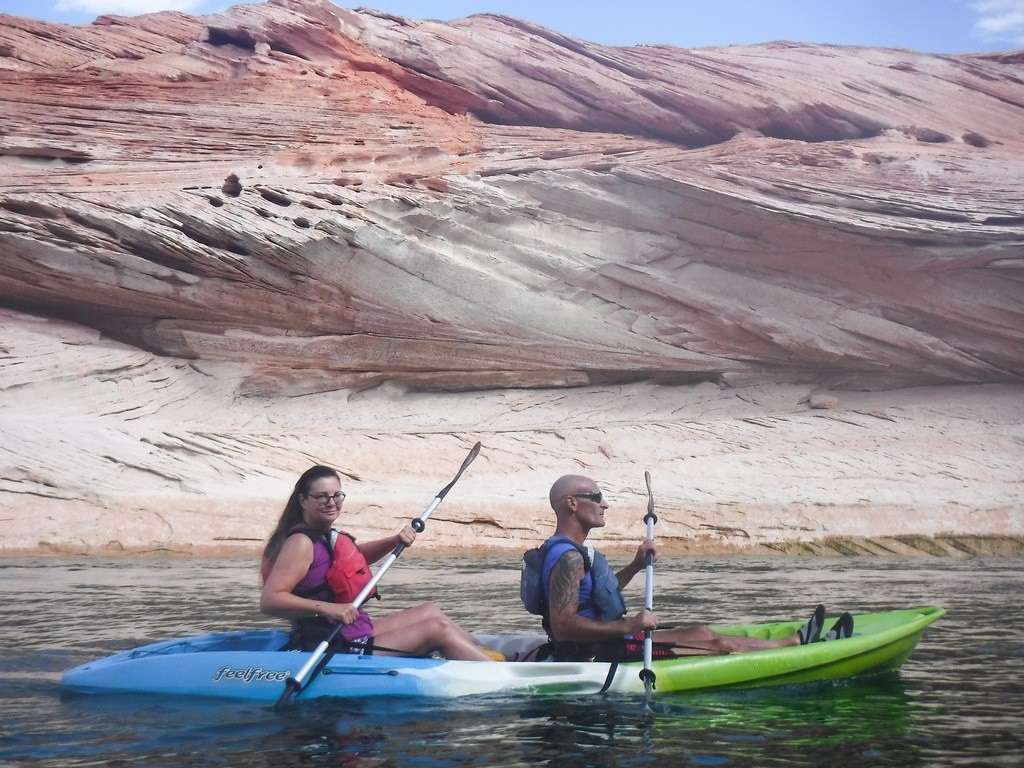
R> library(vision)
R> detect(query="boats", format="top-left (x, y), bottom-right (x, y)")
top-left (48, 602), bottom-right (946, 703)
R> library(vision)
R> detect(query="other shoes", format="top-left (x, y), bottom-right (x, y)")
top-left (821, 612), bottom-right (854, 641)
top-left (797, 604), bottom-right (827, 644)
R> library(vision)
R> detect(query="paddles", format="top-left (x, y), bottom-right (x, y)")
top-left (272, 441), bottom-right (482, 708)
top-left (642, 470), bottom-right (658, 705)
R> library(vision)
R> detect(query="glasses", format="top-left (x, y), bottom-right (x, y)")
top-left (306, 491), bottom-right (346, 506)
top-left (562, 492), bottom-right (602, 503)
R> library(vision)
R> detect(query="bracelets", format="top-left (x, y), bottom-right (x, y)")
top-left (314, 600), bottom-right (321, 616)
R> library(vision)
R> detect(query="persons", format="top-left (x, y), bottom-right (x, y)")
top-left (259, 466), bottom-right (551, 661)
top-left (520, 475), bottom-right (853, 661)
